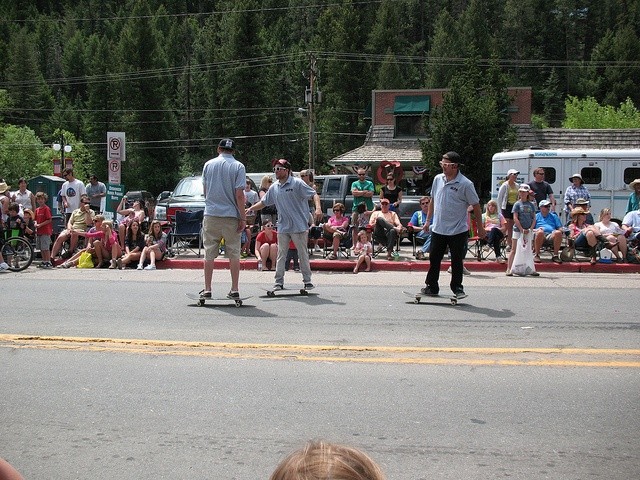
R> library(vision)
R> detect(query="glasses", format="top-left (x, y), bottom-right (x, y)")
top-left (421, 201), bottom-right (429, 204)
top-left (535, 173), bottom-right (545, 175)
top-left (511, 173), bottom-right (518, 176)
top-left (358, 173), bottom-right (366, 176)
top-left (264, 224), bottom-right (274, 228)
top-left (333, 210), bottom-right (341, 212)
top-left (63, 174), bottom-right (69, 176)
top-left (439, 161), bottom-right (455, 167)
top-left (273, 167), bottom-right (286, 172)
top-left (83, 202), bottom-right (91, 204)
top-left (301, 175), bottom-right (309, 177)
top-left (387, 179), bottom-right (394, 181)
top-left (93, 219), bottom-right (100, 222)
top-left (269, 181), bottom-right (273, 183)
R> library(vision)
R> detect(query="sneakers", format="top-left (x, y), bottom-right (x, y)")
top-left (531, 272), bottom-right (540, 276)
top-left (118, 258), bottom-right (122, 269)
top-left (386, 255), bottom-right (395, 261)
top-left (108, 265), bottom-right (114, 268)
top-left (0, 262), bottom-right (9, 272)
top-left (273, 285), bottom-right (283, 290)
top-left (112, 258), bottom-right (118, 269)
top-left (315, 244), bottom-right (321, 252)
top-left (198, 289), bottom-right (211, 299)
top-left (496, 257), bottom-right (506, 264)
top-left (364, 268), bottom-right (371, 271)
top-left (353, 267), bottom-right (358, 274)
top-left (399, 227), bottom-right (407, 235)
top-left (226, 291), bottom-right (239, 300)
top-left (329, 254), bottom-right (337, 260)
top-left (505, 269), bottom-right (513, 276)
top-left (447, 266), bottom-right (452, 273)
top-left (304, 284), bottom-right (314, 290)
top-left (483, 244), bottom-right (490, 252)
top-left (552, 256), bottom-right (562, 263)
top-left (455, 288), bottom-right (465, 298)
top-left (415, 250), bottom-right (426, 260)
top-left (342, 232), bottom-right (347, 238)
top-left (96, 260), bottom-right (105, 268)
top-left (463, 266), bottom-right (470, 275)
top-left (421, 286), bottom-right (438, 295)
top-left (137, 264), bottom-right (143, 270)
top-left (295, 268), bottom-right (300, 271)
top-left (533, 256), bottom-right (542, 263)
top-left (144, 264), bottom-right (156, 270)
top-left (57, 262), bottom-right (67, 268)
top-left (63, 261), bottom-right (73, 268)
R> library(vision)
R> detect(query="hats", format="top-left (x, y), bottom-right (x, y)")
top-left (571, 206), bottom-right (590, 217)
top-left (629, 179), bottom-right (640, 190)
top-left (507, 169), bottom-right (521, 177)
top-left (133, 199), bottom-right (145, 206)
top-left (381, 198), bottom-right (390, 204)
top-left (0, 182), bottom-right (11, 193)
top-left (272, 159), bottom-right (291, 171)
top-left (442, 151), bottom-right (465, 166)
top-left (569, 173), bottom-right (585, 184)
top-left (539, 199), bottom-right (552, 207)
top-left (573, 198), bottom-right (590, 205)
top-left (518, 183), bottom-right (531, 192)
top-left (219, 138), bottom-right (236, 150)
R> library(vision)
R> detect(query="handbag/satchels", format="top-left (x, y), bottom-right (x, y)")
top-left (561, 247), bottom-right (575, 261)
top-left (626, 244), bottom-right (640, 263)
top-left (78, 250), bottom-right (94, 268)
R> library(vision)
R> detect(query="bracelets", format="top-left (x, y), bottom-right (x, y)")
top-left (238, 216), bottom-right (245, 222)
top-left (425, 218), bottom-right (431, 222)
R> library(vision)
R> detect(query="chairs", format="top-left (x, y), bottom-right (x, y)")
top-left (50, 212), bottom-right (97, 258)
top-left (172, 209), bottom-right (205, 258)
top-left (306, 216), bottom-right (416, 256)
top-left (468, 211), bottom-right (639, 262)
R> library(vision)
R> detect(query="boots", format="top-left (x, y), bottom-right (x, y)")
top-left (588, 244), bottom-right (596, 264)
top-left (596, 234), bottom-right (619, 249)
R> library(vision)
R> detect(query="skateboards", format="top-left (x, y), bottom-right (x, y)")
top-left (258, 283), bottom-right (315, 297)
top-left (185, 293), bottom-right (254, 308)
top-left (402, 291), bottom-right (468, 305)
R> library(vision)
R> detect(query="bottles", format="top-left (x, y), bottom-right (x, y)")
top-left (257, 261), bottom-right (263, 272)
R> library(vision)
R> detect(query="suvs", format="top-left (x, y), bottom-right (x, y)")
top-left (126, 190), bottom-right (154, 201)
top-left (154, 175), bottom-right (206, 245)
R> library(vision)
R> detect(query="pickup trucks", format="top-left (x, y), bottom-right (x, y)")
top-left (314, 175), bottom-right (429, 242)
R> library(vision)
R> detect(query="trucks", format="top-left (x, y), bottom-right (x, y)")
top-left (491, 146), bottom-right (640, 222)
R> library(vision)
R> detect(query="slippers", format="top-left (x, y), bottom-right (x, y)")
top-left (262, 267), bottom-right (269, 270)
top-left (270, 267), bottom-right (276, 270)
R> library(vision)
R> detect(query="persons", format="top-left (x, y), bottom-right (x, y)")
top-left (0, 183), bottom-right (11, 225)
top-left (369, 198), bottom-right (408, 261)
top-left (594, 208), bottom-right (630, 264)
top-left (0, 448), bottom-right (25, 480)
top-left (421, 149), bottom-right (486, 299)
top-left (448, 205), bottom-right (473, 275)
top-left (116, 198), bottom-right (149, 250)
top-left (532, 200), bottom-right (563, 264)
top-left (52, 214), bottom-right (105, 270)
top-left (322, 202), bottom-right (349, 260)
top-left (497, 168), bottom-right (519, 252)
top-left (621, 209), bottom-right (640, 243)
top-left (482, 199), bottom-right (508, 264)
top-left (568, 206), bottom-right (607, 264)
top-left (564, 198), bottom-right (596, 226)
top-left (525, 168), bottom-right (556, 213)
top-left (409, 195), bottom-right (432, 260)
top-left (258, 175), bottom-right (277, 225)
top-left (379, 172), bottom-right (403, 216)
top-left (357, 201), bottom-right (377, 230)
top-left (199, 138), bottom-right (246, 299)
top-left (22, 208), bottom-right (35, 243)
top-left (4, 203), bottom-right (27, 269)
top-left (241, 229), bottom-right (253, 257)
top-left (254, 218), bottom-right (278, 272)
top-left (269, 432), bottom-right (384, 480)
top-left (56, 188), bottom-right (65, 216)
top-left (50, 196), bottom-right (94, 266)
top-left (117, 220), bottom-right (144, 269)
top-left (11, 177), bottom-right (36, 213)
top-left (243, 175), bottom-right (259, 257)
top-left (350, 167), bottom-right (375, 250)
top-left (504, 184), bottom-right (539, 277)
top-left (59, 167), bottom-right (87, 248)
top-left (0, 205), bottom-right (12, 272)
top-left (136, 219), bottom-right (168, 270)
top-left (299, 169), bottom-right (321, 252)
top-left (72, 219), bottom-right (121, 269)
top-left (85, 173), bottom-right (106, 214)
top-left (625, 178), bottom-right (640, 213)
top-left (352, 229), bottom-right (374, 274)
top-left (33, 191), bottom-right (52, 268)
top-left (285, 239), bottom-right (300, 273)
top-left (245, 157), bottom-right (323, 289)
top-left (563, 174), bottom-right (592, 225)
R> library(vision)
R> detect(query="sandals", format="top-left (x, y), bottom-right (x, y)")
top-left (36, 261), bottom-right (46, 268)
top-left (247, 252), bottom-right (256, 257)
top-left (43, 262), bottom-right (53, 268)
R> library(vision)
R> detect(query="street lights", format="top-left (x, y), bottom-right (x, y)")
top-left (53, 134), bottom-right (71, 178)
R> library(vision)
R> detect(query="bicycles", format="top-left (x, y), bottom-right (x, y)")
top-left (1, 229), bottom-right (33, 271)
